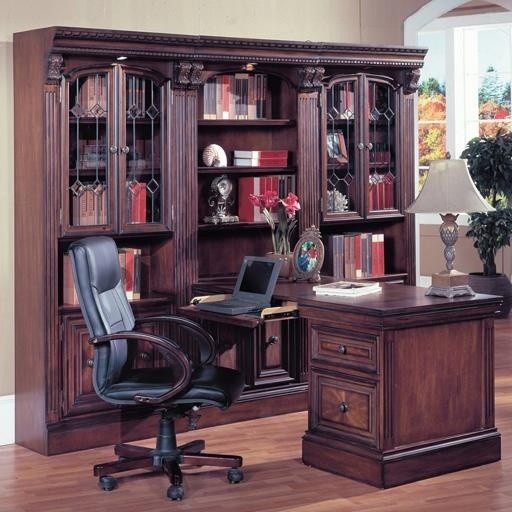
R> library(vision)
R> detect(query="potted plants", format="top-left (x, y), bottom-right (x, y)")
top-left (460, 132), bottom-right (511, 319)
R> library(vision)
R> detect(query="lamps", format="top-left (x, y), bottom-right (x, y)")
top-left (406, 159), bottom-right (496, 296)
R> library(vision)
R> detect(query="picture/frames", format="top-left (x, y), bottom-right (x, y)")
top-left (287, 224), bottom-right (325, 286)
top-left (327, 129), bottom-right (348, 165)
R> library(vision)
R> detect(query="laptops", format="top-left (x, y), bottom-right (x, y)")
top-left (199, 256), bottom-right (283, 315)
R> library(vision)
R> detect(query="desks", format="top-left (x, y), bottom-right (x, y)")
top-left (214, 281), bottom-right (504, 489)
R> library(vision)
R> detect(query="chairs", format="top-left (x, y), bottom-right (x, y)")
top-left (71, 236), bottom-right (246, 500)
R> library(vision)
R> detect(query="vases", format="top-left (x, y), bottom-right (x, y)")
top-left (267, 252), bottom-right (293, 277)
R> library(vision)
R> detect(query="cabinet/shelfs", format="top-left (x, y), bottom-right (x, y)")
top-left (13, 26), bottom-right (427, 455)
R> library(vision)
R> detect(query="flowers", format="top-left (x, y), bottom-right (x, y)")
top-left (247, 190), bottom-right (300, 254)
